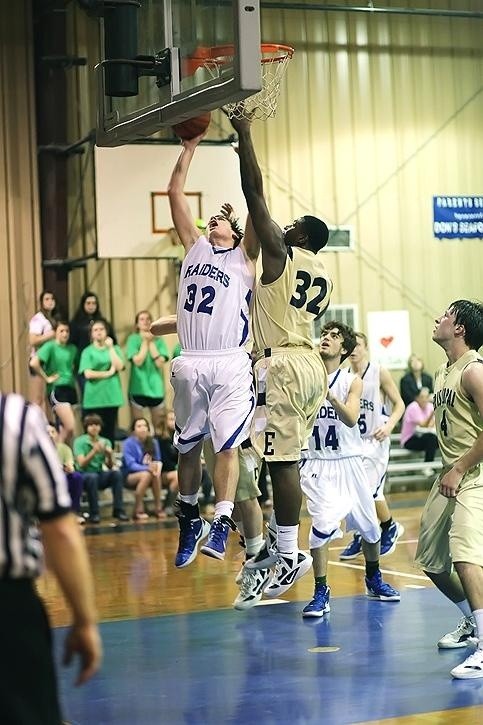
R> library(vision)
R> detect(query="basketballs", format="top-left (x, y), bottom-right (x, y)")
top-left (171, 112), bottom-right (210, 139)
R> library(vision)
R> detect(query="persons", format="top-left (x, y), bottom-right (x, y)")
top-left (31, 290), bottom-right (212, 524)
top-left (339, 332), bottom-right (405, 560)
top-left (166, 128), bottom-right (262, 568)
top-left (400, 354), bottom-right (434, 407)
top-left (1, 391), bottom-right (103, 725)
top-left (413, 300), bottom-right (483, 680)
top-left (218, 99), bottom-right (333, 609)
top-left (401, 387), bottom-right (439, 476)
top-left (301, 322), bottom-right (401, 617)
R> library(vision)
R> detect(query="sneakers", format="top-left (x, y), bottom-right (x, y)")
top-left (422, 469), bottom-right (435, 477)
top-left (200, 521), bottom-right (230, 561)
top-left (340, 532), bottom-right (365, 561)
top-left (77, 507), bottom-right (174, 526)
top-left (232, 520), bottom-right (314, 611)
top-left (436, 613), bottom-right (479, 651)
top-left (174, 508), bottom-right (211, 568)
top-left (302, 584), bottom-right (332, 619)
top-left (448, 645), bottom-right (483, 680)
top-left (377, 520), bottom-right (404, 556)
top-left (363, 568), bottom-right (402, 601)
